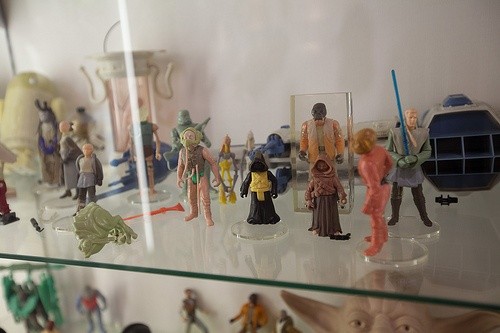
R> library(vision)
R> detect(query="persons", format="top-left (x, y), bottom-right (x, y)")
top-left (351, 127), bottom-right (394, 256)
top-left (299, 102), bottom-right (344, 182)
top-left (118, 107), bottom-right (162, 197)
top-left (179, 288), bottom-right (218, 333)
top-left (72, 144), bottom-right (104, 217)
top-left (239, 151), bottom-right (281, 224)
top-left (176, 127), bottom-right (221, 226)
top-left (387, 109), bottom-right (432, 227)
top-left (304, 152), bottom-right (347, 236)
top-left (230, 293), bottom-right (268, 333)
top-left (58, 120), bottom-right (84, 201)
top-left (275, 310), bottom-right (293, 333)
top-left (78, 285), bottom-right (108, 333)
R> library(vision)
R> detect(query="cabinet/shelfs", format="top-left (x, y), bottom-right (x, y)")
top-left (0, 146), bottom-right (500, 333)
